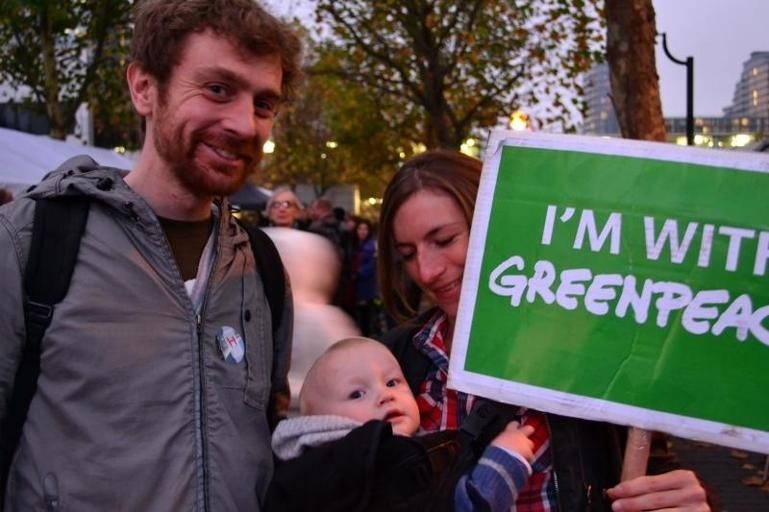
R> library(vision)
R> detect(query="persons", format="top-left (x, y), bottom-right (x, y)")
top-left (240, 185), bottom-right (384, 417)
top-left (267, 335), bottom-right (536, 511)
top-left (377, 149), bottom-right (716, 512)
top-left (1, 0), bottom-right (304, 511)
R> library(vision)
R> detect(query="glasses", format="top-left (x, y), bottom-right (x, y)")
top-left (270, 200), bottom-right (295, 208)
